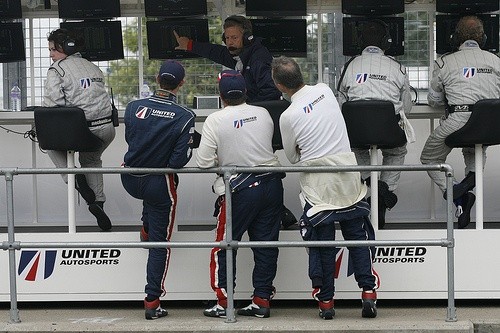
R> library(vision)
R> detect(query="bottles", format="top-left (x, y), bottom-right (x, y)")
top-left (10, 82), bottom-right (21, 112)
top-left (139, 82), bottom-right (150, 100)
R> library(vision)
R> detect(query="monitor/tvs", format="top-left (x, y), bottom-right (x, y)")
top-left (146, 19), bottom-right (209, 59)
top-left (248, 19), bottom-right (307, 57)
top-left (246, 0), bottom-right (306, 17)
top-left (0, 0), bottom-right (22, 19)
top-left (436, 14), bottom-right (500, 54)
top-left (0, 23), bottom-right (25, 63)
top-left (60, 21), bottom-right (123, 60)
top-left (144, 0), bottom-right (207, 16)
top-left (436, 0), bottom-right (500, 14)
top-left (58, 0), bottom-right (120, 20)
top-left (342, 17), bottom-right (405, 56)
top-left (342, 0), bottom-right (404, 15)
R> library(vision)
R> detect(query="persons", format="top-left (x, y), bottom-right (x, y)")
top-left (173, 15), bottom-right (291, 153)
top-left (271, 56), bottom-right (381, 319)
top-left (43, 27), bottom-right (115, 230)
top-left (195, 71), bottom-right (286, 318)
top-left (121, 59), bottom-right (197, 320)
top-left (336, 20), bottom-right (413, 210)
top-left (419, 16), bottom-right (500, 229)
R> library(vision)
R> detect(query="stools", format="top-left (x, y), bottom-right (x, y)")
top-left (341, 100), bottom-right (407, 229)
top-left (34, 107), bottom-right (106, 233)
top-left (249, 99), bottom-right (290, 151)
top-left (445, 98), bottom-right (500, 229)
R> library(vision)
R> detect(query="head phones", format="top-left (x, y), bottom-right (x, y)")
top-left (222, 15), bottom-right (256, 47)
top-left (62, 30), bottom-right (75, 56)
top-left (357, 19), bottom-right (393, 49)
top-left (449, 24), bottom-right (487, 49)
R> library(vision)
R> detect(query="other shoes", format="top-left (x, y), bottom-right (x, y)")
top-left (75, 174), bottom-right (96, 204)
top-left (281, 209), bottom-right (297, 228)
top-left (88, 203), bottom-right (112, 231)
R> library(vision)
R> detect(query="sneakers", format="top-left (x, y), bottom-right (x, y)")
top-left (318, 301), bottom-right (335, 320)
top-left (452, 191), bottom-right (476, 228)
top-left (236, 297), bottom-right (270, 318)
top-left (443, 173), bottom-right (476, 200)
top-left (204, 304), bottom-right (227, 318)
top-left (140, 226), bottom-right (149, 242)
top-left (362, 290), bottom-right (377, 319)
top-left (378, 180), bottom-right (398, 210)
top-left (144, 296), bottom-right (168, 320)
top-left (378, 202), bottom-right (386, 229)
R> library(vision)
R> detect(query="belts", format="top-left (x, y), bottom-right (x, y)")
top-left (446, 103), bottom-right (475, 114)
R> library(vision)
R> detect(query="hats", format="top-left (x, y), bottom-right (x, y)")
top-left (159, 60), bottom-right (185, 83)
top-left (217, 69), bottom-right (246, 100)
